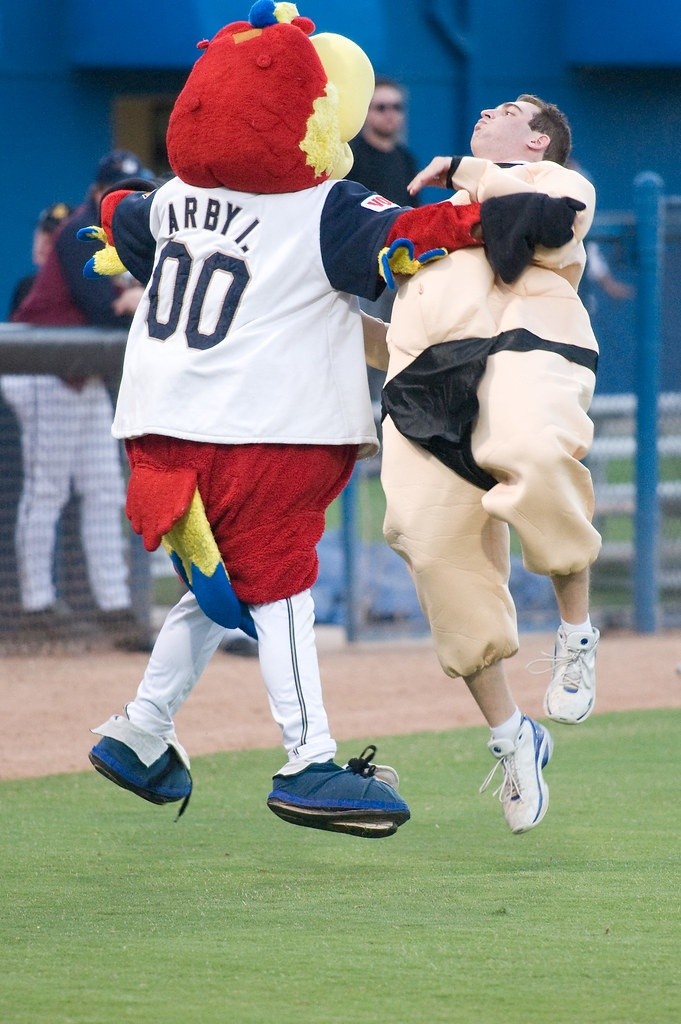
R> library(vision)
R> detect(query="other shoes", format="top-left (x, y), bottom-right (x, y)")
top-left (98, 609), bottom-right (147, 652)
top-left (23, 608), bottom-right (91, 637)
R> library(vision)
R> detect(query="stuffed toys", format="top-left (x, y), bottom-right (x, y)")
top-left (75, 1), bottom-right (589, 838)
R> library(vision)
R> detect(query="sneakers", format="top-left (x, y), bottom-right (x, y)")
top-left (479, 713), bottom-right (553, 834)
top-left (525, 625), bottom-right (600, 724)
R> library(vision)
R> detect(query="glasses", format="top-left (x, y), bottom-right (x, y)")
top-left (375, 103), bottom-right (401, 112)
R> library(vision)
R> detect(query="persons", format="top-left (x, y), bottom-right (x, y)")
top-left (565, 161), bottom-right (629, 321)
top-left (359, 91), bottom-right (603, 836)
top-left (346, 81), bottom-right (425, 402)
top-left (1, 146), bottom-right (263, 654)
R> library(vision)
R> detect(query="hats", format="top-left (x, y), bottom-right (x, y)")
top-left (33, 202), bottom-right (75, 232)
top-left (96, 150), bottom-right (155, 188)
